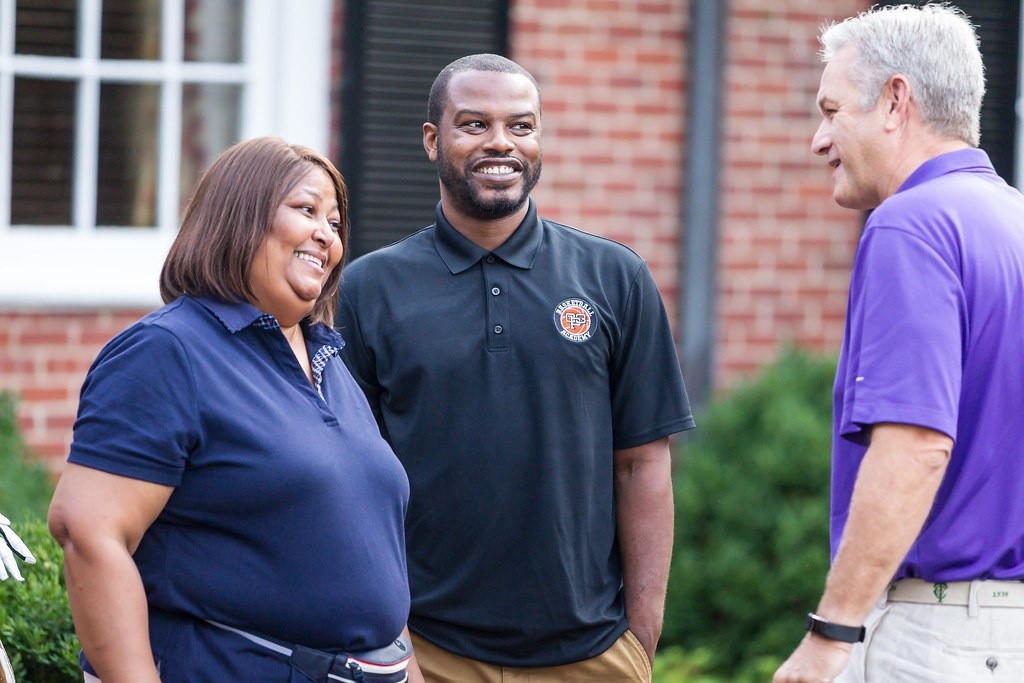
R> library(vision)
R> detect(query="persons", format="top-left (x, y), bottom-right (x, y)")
top-left (329, 54), bottom-right (676, 682)
top-left (774, 5), bottom-right (1023, 683)
top-left (46, 137), bottom-right (412, 682)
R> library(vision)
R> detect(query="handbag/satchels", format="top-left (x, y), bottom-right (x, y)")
top-left (209, 616), bottom-right (411, 683)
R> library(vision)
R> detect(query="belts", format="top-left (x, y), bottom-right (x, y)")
top-left (887, 577), bottom-right (1024, 608)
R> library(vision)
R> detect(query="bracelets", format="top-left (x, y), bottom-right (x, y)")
top-left (806, 613), bottom-right (867, 644)
